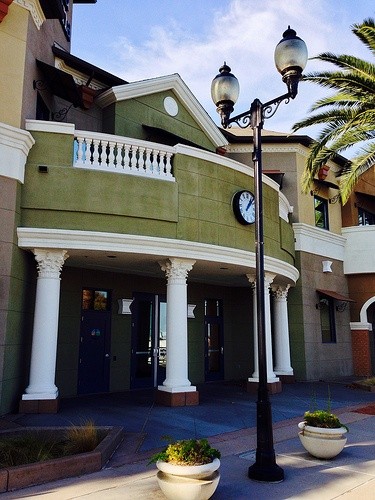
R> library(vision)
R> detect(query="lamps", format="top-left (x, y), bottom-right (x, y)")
top-left (336, 301), bottom-right (349, 312)
top-left (315, 298), bottom-right (329, 311)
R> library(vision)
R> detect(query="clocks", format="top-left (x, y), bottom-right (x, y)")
top-left (232, 190), bottom-right (256, 225)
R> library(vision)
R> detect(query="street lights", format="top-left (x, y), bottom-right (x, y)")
top-left (209, 22), bottom-right (310, 481)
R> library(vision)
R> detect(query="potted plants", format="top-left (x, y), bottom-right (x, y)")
top-left (298, 385), bottom-right (349, 460)
top-left (146, 436), bottom-right (221, 500)
